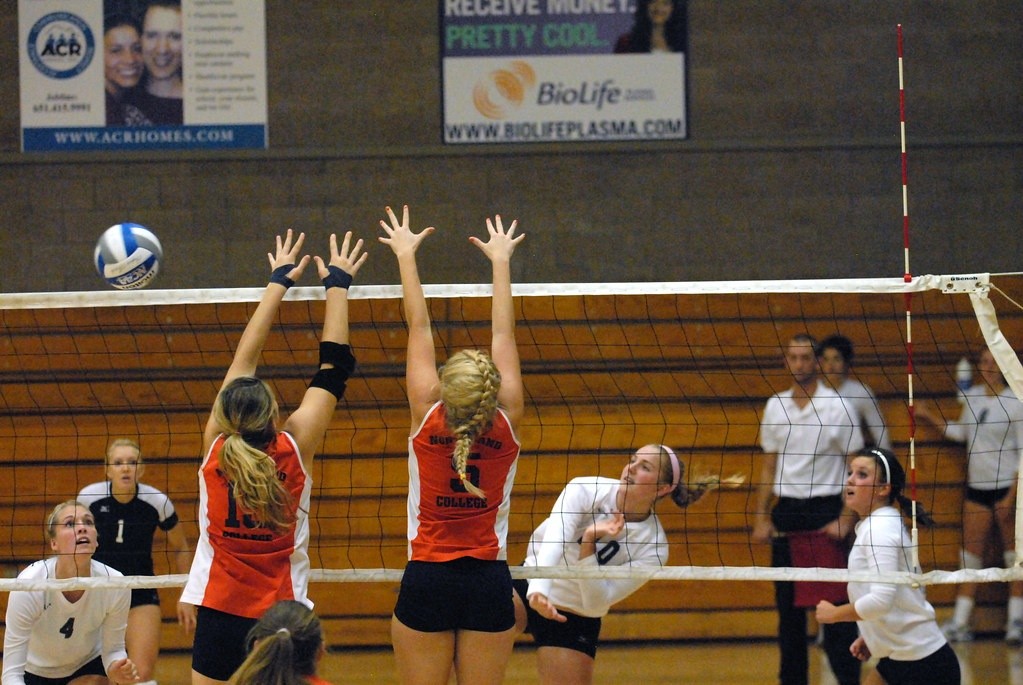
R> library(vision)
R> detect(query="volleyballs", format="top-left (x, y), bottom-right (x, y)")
top-left (93, 221), bottom-right (165, 290)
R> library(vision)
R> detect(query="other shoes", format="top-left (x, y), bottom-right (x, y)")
top-left (940, 621), bottom-right (974, 642)
top-left (1005, 621), bottom-right (1023, 643)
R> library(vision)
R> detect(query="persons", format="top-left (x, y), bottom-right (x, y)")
top-left (103, 0), bottom-right (183, 126)
top-left (228, 600), bottom-right (338, 685)
top-left (750, 332), bottom-right (865, 685)
top-left (914, 344), bottom-right (1023, 647)
top-left (512, 444), bottom-right (746, 685)
top-left (816, 447), bottom-right (962, 685)
top-left (1, 501), bottom-right (140, 685)
top-left (815, 335), bottom-right (891, 451)
top-left (179, 228), bottom-right (368, 685)
top-left (76, 439), bottom-right (196, 685)
top-left (378, 204), bottom-right (524, 684)
top-left (612, 0), bottom-right (686, 53)
